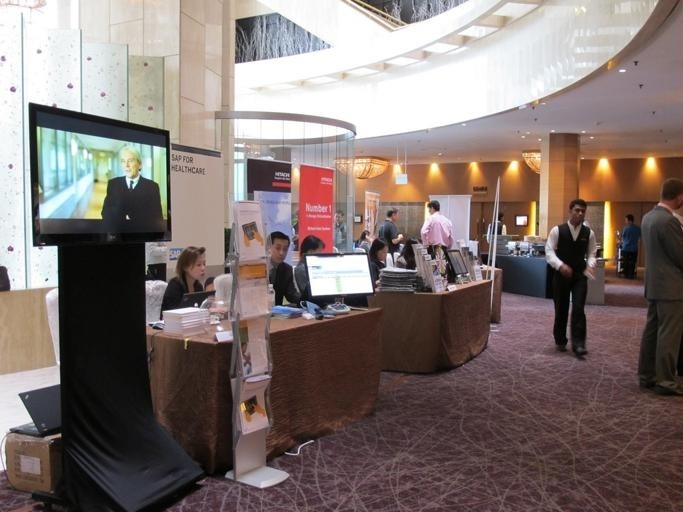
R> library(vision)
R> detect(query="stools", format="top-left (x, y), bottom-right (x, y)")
top-left (637, 267), bottom-right (646, 281)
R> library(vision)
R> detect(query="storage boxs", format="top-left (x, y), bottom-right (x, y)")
top-left (5, 432), bottom-right (62, 495)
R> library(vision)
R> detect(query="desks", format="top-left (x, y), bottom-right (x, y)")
top-left (147, 308), bottom-right (382, 477)
top-left (480, 265), bottom-right (503, 322)
top-left (596, 249), bottom-right (603, 257)
top-left (569, 258), bottom-right (608, 305)
top-left (481, 253), bottom-right (553, 298)
top-left (367, 280), bottom-right (492, 375)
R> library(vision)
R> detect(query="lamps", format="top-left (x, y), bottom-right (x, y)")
top-left (522, 152), bottom-right (541, 173)
top-left (334, 158), bottom-right (390, 180)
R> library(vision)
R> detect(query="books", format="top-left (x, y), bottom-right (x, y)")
top-left (161, 305), bottom-right (211, 339)
top-left (374, 266), bottom-right (426, 294)
top-left (412, 242), bottom-right (446, 293)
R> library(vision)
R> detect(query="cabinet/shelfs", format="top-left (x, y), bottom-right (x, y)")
top-left (224, 193), bottom-right (289, 488)
top-left (617, 243), bottom-right (636, 277)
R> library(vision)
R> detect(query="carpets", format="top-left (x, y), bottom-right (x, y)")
top-left (0, 270), bottom-right (683, 512)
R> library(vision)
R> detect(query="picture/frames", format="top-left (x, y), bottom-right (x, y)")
top-left (515, 215), bottom-right (527, 226)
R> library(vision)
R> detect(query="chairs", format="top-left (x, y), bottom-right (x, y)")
top-left (146, 280), bottom-right (169, 323)
top-left (46, 288), bottom-right (60, 366)
top-left (394, 252), bottom-right (400, 263)
top-left (386, 252), bottom-right (393, 267)
top-left (213, 274), bottom-right (233, 308)
top-left (146, 263), bottom-right (168, 282)
top-left (457, 240), bottom-right (465, 248)
top-left (353, 248), bottom-right (365, 253)
top-left (468, 240), bottom-right (478, 257)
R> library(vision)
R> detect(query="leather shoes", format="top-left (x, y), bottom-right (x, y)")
top-left (556, 342), bottom-right (567, 350)
top-left (572, 342), bottom-right (588, 355)
top-left (641, 377), bottom-right (679, 395)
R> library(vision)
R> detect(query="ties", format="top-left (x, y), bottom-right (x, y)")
top-left (270, 267), bottom-right (277, 285)
top-left (127, 180), bottom-right (135, 192)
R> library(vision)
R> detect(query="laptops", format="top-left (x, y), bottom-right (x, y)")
top-left (149, 290), bottom-right (216, 331)
top-left (9, 384), bottom-right (61, 437)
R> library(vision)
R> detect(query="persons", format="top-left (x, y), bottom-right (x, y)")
top-left (334, 210), bottom-right (346, 252)
top-left (265, 232), bottom-right (303, 308)
top-left (543, 200), bottom-right (598, 356)
top-left (379, 209), bottom-right (403, 266)
top-left (620, 214), bottom-right (642, 278)
top-left (355, 230), bottom-right (371, 254)
top-left (486, 212), bottom-right (508, 245)
top-left (100, 147), bottom-right (164, 224)
top-left (294, 235), bottom-right (336, 308)
top-left (368, 238), bottom-right (389, 293)
top-left (420, 201), bottom-right (454, 260)
top-left (637, 178), bottom-right (683, 397)
top-left (674, 197), bottom-right (683, 225)
top-left (159, 247), bottom-right (209, 320)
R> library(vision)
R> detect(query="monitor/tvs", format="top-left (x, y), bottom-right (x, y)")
top-left (26, 102), bottom-right (171, 246)
top-left (303, 253), bottom-right (376, 311)
top-left (514, 216), bottom-right (528, 227)
top-left (445, 247), bottom-right (470, 283)
